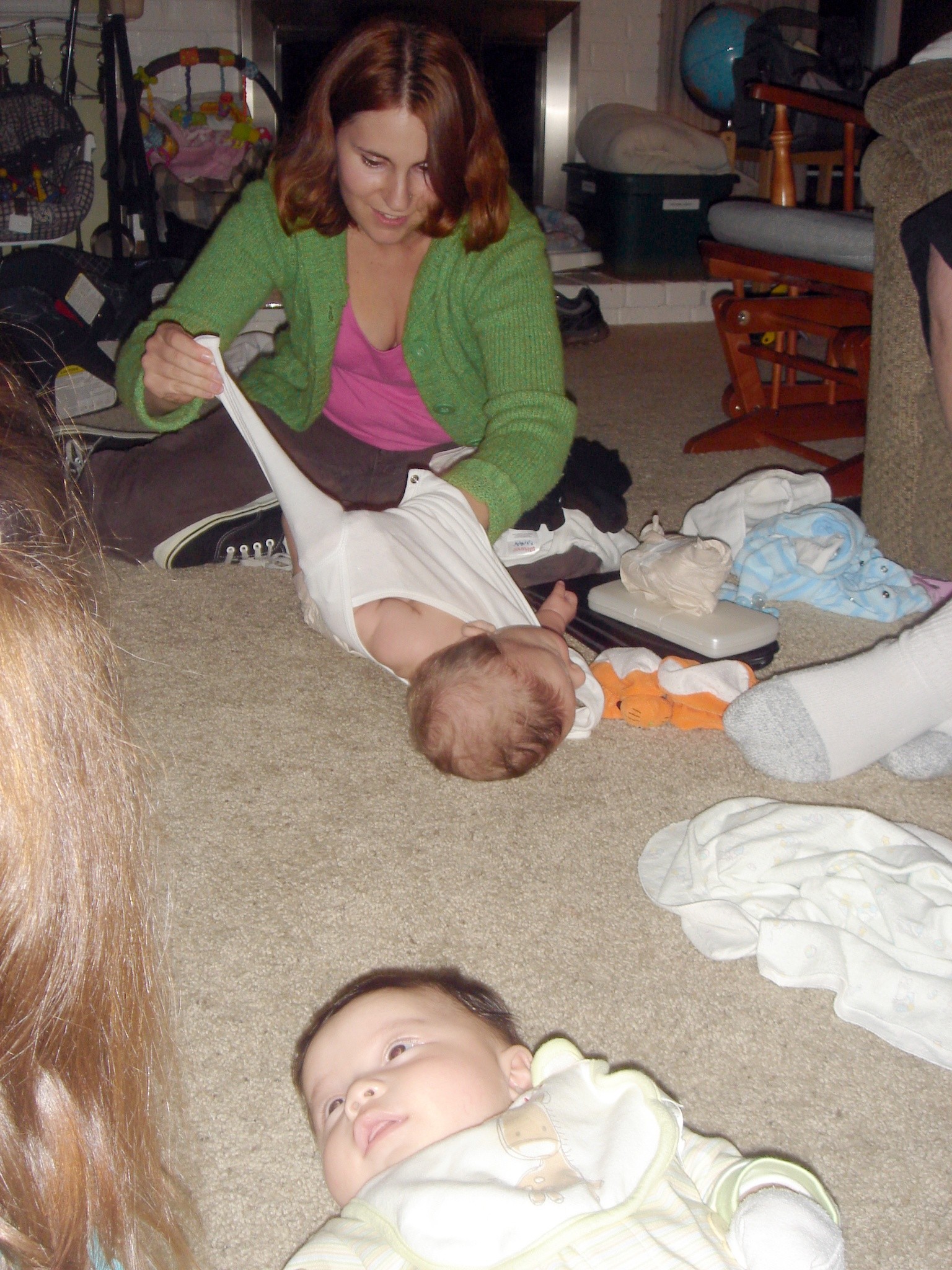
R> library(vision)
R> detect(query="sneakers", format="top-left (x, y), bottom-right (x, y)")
top-left (51, 424), bottom-right (157, 470)
top-left (550, 288), bottom-right (608, 341)
top-left (152, 492), bottom-right (294, 571)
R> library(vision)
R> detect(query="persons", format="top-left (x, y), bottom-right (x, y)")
top-left (714, 188), bottom-right (951, 791)
top-left (276, 472), bottom-right (587, 783)
top-left (1, 379), bottom-right (197, 1268)
top-left (290, 966), bottom-right (843, 1268)
top-left (83, 25), bottom-right (602, 574)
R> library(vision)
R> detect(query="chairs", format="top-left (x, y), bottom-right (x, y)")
top-left (683, 82), bottom-right (882, 499)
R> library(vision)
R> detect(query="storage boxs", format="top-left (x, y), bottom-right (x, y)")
top-left (559, 162), bottom-right (741, 281)
